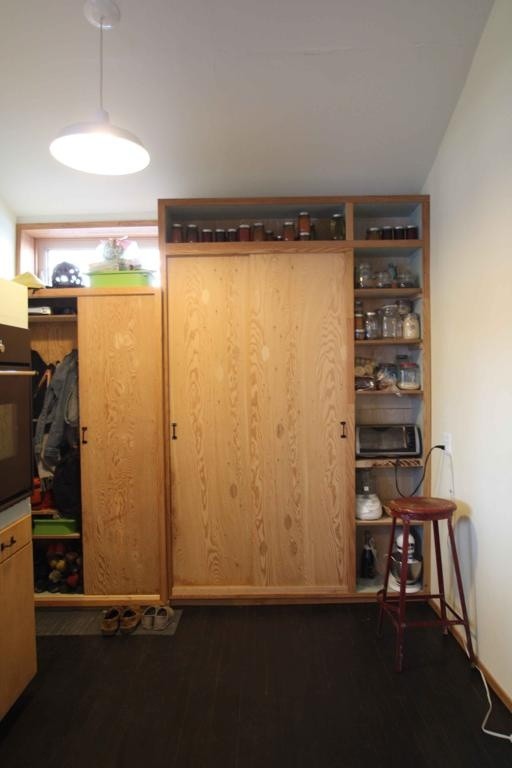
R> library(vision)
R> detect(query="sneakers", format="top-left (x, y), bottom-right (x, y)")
top-left (153, 605), bottom-right (175, 632)
top-left (119, 604), bottom-right (143, 635)
top-left (99, 606), bottom-right (125, 638)
top-left (141, 604), bottom-right (161, 631)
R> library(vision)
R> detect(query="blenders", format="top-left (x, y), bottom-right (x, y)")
top-left (385, 530), bottom-right (424, 593)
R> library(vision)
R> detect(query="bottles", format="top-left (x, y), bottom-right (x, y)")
top-left (356, 261), bottom-right (421, 392)
top-left (359, 532), bottom-right (376, 580)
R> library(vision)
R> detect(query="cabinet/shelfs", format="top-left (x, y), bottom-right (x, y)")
top-left (2, 514), bottom-right (40, 720)
top-left (157, 194), bottom-right (431, 609)
top-left (29, 286), bottom-right (164, 610)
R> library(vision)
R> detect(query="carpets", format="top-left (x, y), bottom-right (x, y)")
top-left (36, 606), bottom-right (184, 638)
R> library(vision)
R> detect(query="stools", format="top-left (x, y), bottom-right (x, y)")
top-left (375, 494), bottom-right (480, 674)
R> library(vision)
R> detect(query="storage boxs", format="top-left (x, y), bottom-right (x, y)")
top-left (87, 268), bottom-right (153, 287)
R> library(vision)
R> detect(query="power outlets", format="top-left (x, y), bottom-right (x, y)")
top-left (440, 432), bottom-right (453, 457)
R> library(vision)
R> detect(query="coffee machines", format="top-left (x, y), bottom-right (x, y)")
top-left (356, 472), bottom-right (382, 520)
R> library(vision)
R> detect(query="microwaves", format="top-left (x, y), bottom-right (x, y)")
top-left (355, 423), bottom-right (421, 457)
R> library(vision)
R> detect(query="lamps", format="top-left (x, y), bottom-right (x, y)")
top-left (46, 0), bottom-right (152, 178)
